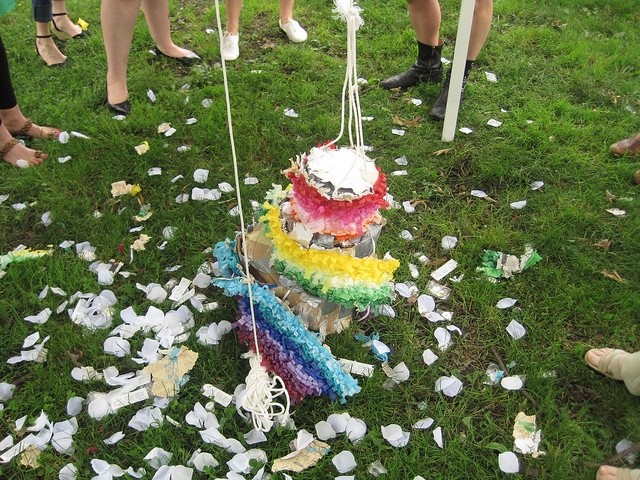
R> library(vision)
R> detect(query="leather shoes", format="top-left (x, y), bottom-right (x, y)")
top-left (155, 44), bottom-right (201, 65)
top-left (105, 81), bottom-right (132, 116)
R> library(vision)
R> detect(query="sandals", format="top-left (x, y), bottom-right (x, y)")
top-left (51, 13), bottom-right (91, 40)
top-left (35, 35), bottom-right (75, 68)
top-left (597, 466), bottom-right (640, 479)
top-left (585, 347), bottom-right (632, 381)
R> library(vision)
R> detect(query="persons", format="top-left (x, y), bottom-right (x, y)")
top-left (584, 346), bottom-right (640, 480)
top-left (34, 0), bottom-right (88, 70)
top-left (220, 0), bottom-right (307, 62)
top-left (609, 132), bottom-right (639, 184)
top-left (0, 37), bottom-right (61, 169)
top-left (99, 0), bottom-right (202, 116)
top-left (378, 0), bottom-right (493, 121)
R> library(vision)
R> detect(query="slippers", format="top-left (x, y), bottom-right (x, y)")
top-left (9, 118), bottom-right (61, 141)
top-left (0, 138), bottom-right (48, 168)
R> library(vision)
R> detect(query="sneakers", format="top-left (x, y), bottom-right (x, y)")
top-left (610, 132), bottom-right (640, 158)
top-left (223, 30), bottom-right (239, 60)
top-left (279, 18), bottom-right (307, 43)
top-left (634, 171), bottom-right (640, 183)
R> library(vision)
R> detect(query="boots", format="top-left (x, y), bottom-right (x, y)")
top-left (379, 37), bottom-right (443, 89)
top-left (429, 59), bottom-right (474, 120)
